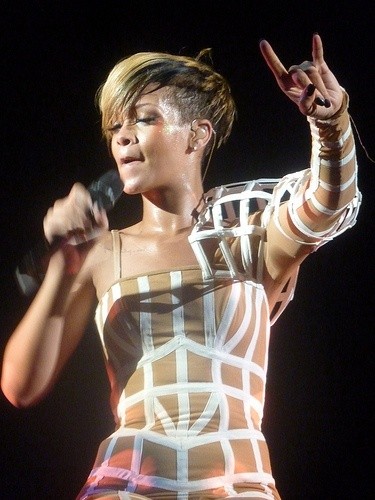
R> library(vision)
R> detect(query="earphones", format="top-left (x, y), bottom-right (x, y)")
top-left (192, 128), bottom-right (208, 147)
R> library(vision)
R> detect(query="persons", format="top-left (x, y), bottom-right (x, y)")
top-left (0, 34), bottom-right (364, 499)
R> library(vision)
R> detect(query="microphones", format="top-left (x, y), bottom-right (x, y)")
top-left (18, 173), bottom-right (123, 279)
